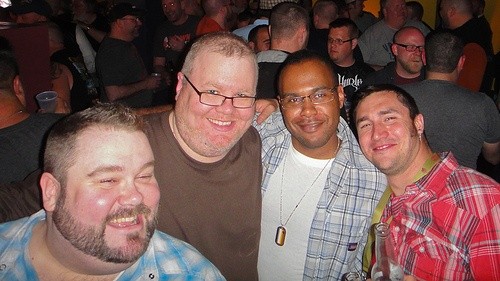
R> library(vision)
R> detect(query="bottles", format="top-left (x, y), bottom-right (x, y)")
top-left (370, 222), bottom-right (404, 281)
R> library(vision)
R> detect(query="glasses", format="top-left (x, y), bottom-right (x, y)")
top-left (327, 38), bottom-right (354, 45)
top-left (121, 16), bottom-right (140, 21)
top-left (396, 43), bottom-right (425, 52)
top-left (183, 72), bottom-right (257, 109)
top-left (279, 86), bottom-right (337, 110)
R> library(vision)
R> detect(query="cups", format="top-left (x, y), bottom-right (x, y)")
top-left (35, 91), bottom-right (58, 113)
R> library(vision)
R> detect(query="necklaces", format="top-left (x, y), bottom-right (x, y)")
top-left (275, 138), bottom-right (340, 246)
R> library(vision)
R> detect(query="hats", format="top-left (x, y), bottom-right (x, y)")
top-left (108, 3), bottom-right (145, 21)
top-left (13, 0), bottom-right (53, 19)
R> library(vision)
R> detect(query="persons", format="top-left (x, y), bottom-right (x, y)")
top-left (334, 0), bottom-right (376, 36)
top-left (348, 82), bottom-right (500, 281)
top-left (358, 0), bottom-right (431, 72)
top-left (328, 18), bottom-right (376, 125)
top-left (364, 26), bottom-right (426, 85)
top-left (306, 0), bottom-right (342, 55)
top-left (0, 50), bottom-right (67, 219)
top-left (407, 1), bottom-right (434, 32)
top-left (439, 0), bottom-right (494, 94)
top-left (131, 49), bottom-right (387, 281)
top-left (397, 28), bottom-right (500, 169)
top-left (8, 0), bottom-right (260, 114)
top-left (471, 0), bottom-right (490, 41)
top-left (138, 32), bottom-right (277, 281)
top-left (0, 106), bottom-right (231, 281)
top-left (245, 1), bottom-right (309, 99)
